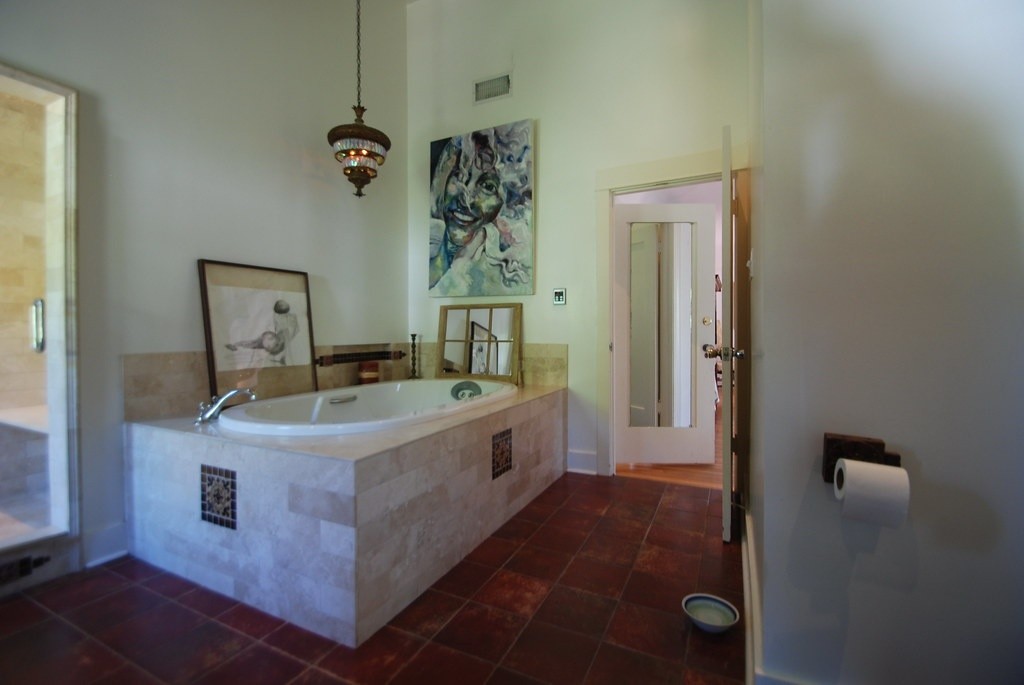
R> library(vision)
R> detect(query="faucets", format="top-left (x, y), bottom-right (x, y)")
top-left (195, 387), bottom-right (257, 424)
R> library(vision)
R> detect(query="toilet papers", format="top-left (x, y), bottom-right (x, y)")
top-left (832, 457), bottom-right (911, 531)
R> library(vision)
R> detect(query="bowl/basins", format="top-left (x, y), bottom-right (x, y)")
top-left (681, 592), bottom-right (739, 631)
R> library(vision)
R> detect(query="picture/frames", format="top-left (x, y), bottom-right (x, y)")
top-left (469, 321), bottom-right (498, 374)
top-left (197, 258), bottom-right (320, 410)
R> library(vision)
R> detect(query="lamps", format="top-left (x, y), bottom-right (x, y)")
top-left (327, 0), bottom-right (391, 199)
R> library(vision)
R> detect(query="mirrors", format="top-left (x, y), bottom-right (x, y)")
top-left (432, 303), bottom-right (523, 385)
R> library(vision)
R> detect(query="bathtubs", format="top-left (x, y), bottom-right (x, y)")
top-left (132, 378), bottom-right (565, 649)
